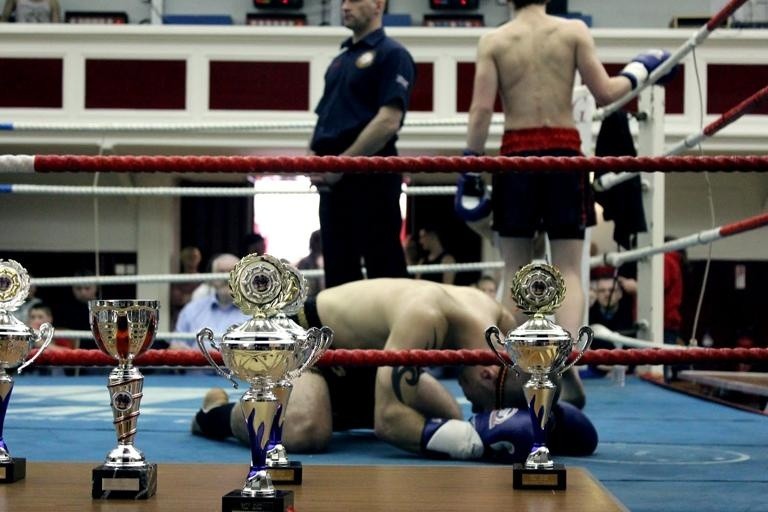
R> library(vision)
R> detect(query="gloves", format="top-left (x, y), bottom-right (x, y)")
top-left (420, 409), bottom-right (535, 465)
top-left (620, 45), bottom-right (672, 91)
top-left (547, 400), bottom-right (597, 456)
top-left (456, 149), bottom-right (492, 222)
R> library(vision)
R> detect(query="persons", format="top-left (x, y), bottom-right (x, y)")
top-left (192, 278), bottom-right (597, 465)
top-left (303, 0), bottom-right (415, 289)
top-left (454, 0), bottom-right (671, 380)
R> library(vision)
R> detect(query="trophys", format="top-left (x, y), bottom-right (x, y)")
top-left (88, 299), bottom-right (160, 500)
top-left (197, 253), bottom-right (324, 512)
top-left (484, 261), bottom-right (594, 491)
top-left (250, 262), bottom-right (335, 486)
top-left (0, 258), bottom-right (54, 483)
